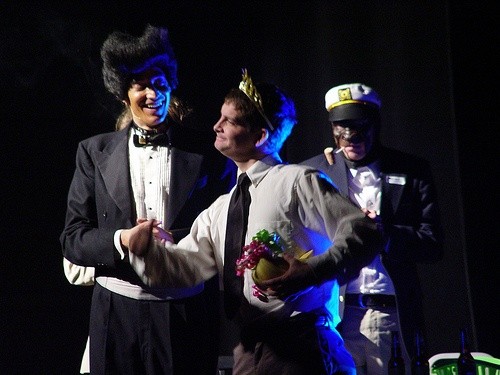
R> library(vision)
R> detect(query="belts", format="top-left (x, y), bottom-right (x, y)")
top-left (346, 293), bottom-right (397, 313)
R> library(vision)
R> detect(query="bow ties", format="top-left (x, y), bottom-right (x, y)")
top-left (133, 133), bottom-right (170, 148)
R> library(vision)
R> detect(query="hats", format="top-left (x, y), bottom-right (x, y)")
top-left (324, 83), bottom-right (384, 122)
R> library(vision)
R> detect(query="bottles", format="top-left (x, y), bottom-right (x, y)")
top-left (388, 330), bottom-right (405, 375)
top-left (457, 328), bottom-right (476, 375)
top-left (411, 326), bottom-right (429, 375)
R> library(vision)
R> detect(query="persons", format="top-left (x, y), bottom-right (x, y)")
top-left (59, 24), bottom-right (236, 375)
top-left (138, 68), bottom-right (380, 375)
top-left (295, 83), bottom-right (439, 375)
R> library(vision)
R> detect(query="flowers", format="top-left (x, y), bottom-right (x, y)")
top-left (233, 229), bottom-right (306, 302)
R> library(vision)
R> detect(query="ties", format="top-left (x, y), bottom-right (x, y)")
top-left (224, 172), bottom-right (251, 302)
